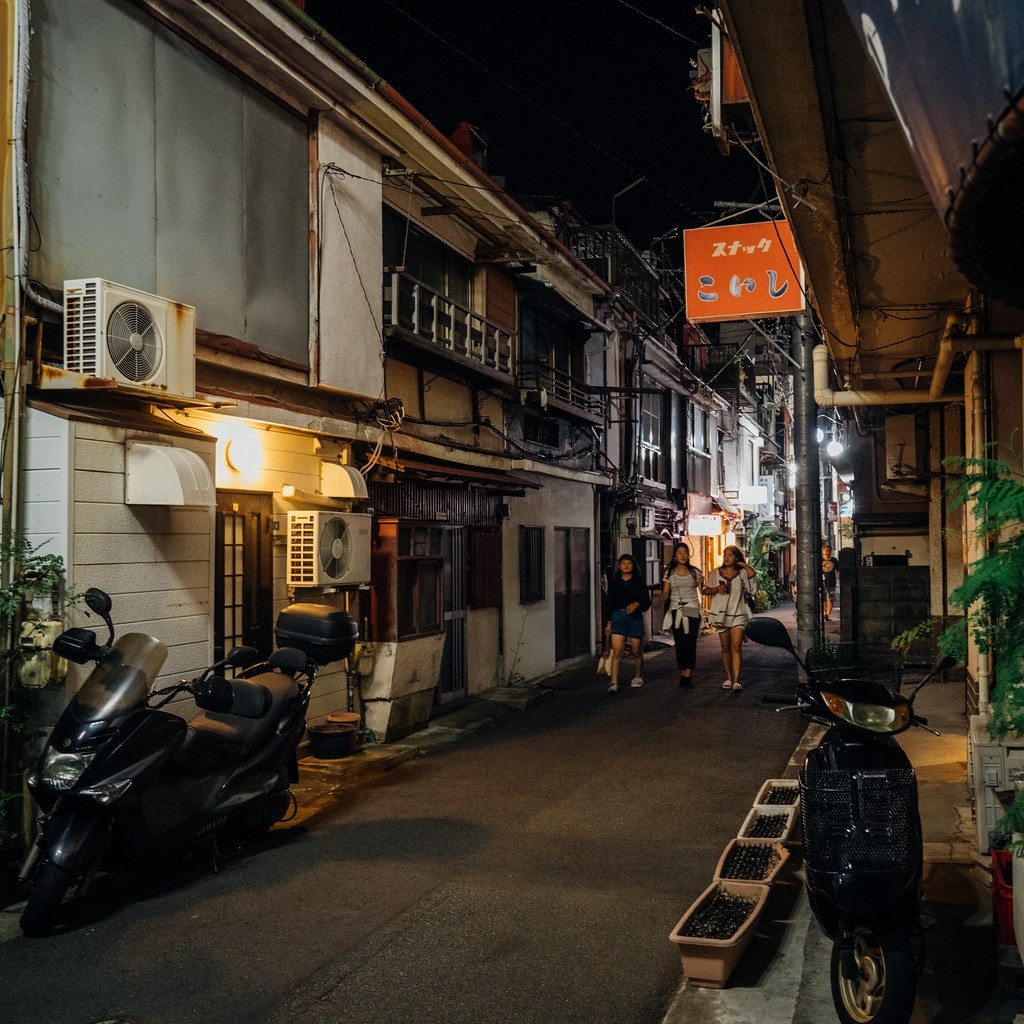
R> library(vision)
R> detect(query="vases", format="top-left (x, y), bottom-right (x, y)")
top-left (669, 778), bottom-right (802, 991)
top-left (307, 711), bottom-right (361, 759)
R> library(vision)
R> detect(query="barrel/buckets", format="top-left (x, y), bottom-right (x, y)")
top-left (326, 712), bottom-right (361, 745)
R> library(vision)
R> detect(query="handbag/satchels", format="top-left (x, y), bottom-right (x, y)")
top-left (597, 633), bottom-right (613, 676)
top-left (682, 607), bottom-right (699, 619)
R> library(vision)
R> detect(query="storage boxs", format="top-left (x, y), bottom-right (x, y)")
top-left (990, 850), bottom-right (1017, 942)
top-left (871, 555), bottom-right (908, 566)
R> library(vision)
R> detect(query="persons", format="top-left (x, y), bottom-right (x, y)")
top-left (661, 543), bottom-right (758, 690)
top-left (605, 554), bottom-right (651, 692)
top-left (789, 565), bottom-right (797, 603)
top-left (822, 545), bottom-right (839, 622)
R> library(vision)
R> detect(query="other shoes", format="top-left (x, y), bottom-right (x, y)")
top-left (824, 614), bottom-right (831, 621)
top-left (733, 683), bottom-right (743, 691)
top-left (631, 678), bottom-right (643, 687)
top-left (722, 680), bottom-right (733, 689)
top-left (608, 683), bottom-right (619, 691)
top-left (679, 676), bottom-right (692, 689)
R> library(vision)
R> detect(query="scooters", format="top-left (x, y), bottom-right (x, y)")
top-left (742, 617), bottom-right (958, 1024)
top-left (18, 588), bottom-right (362, 934)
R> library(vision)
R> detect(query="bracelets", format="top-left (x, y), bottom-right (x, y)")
top-left (607, 623), bottom-right (612, 624)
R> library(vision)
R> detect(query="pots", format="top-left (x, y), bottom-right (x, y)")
top-left (308, 723), bottom-right (373, 759)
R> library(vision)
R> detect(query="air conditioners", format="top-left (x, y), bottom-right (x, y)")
top-left (63, 277), bottom-right (197, 398)
top-left (287, 510), bottom-right (371, 585)
top-left (967, 715), bottom-right (1024, 852)
